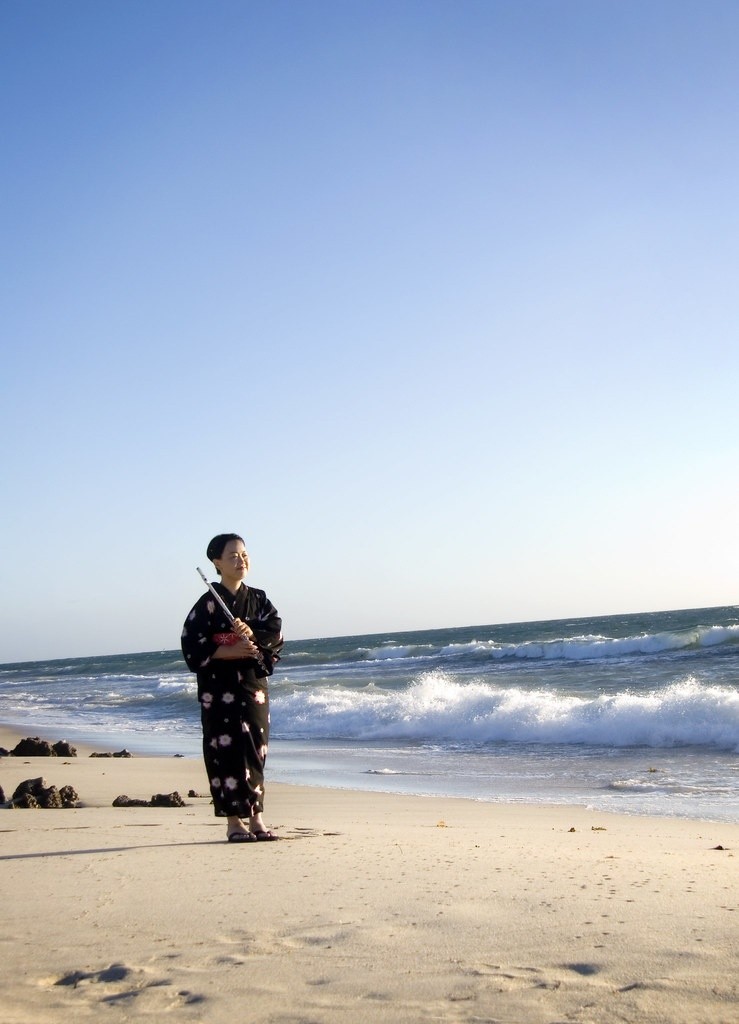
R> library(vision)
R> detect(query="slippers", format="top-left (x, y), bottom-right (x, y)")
top-left (256, 830), bottom-right (279, 841)
top-left (229, 832), bottom-right (257, 842)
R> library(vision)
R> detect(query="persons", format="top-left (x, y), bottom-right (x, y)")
top-left (181, 534), bottom-right (285, 842)
